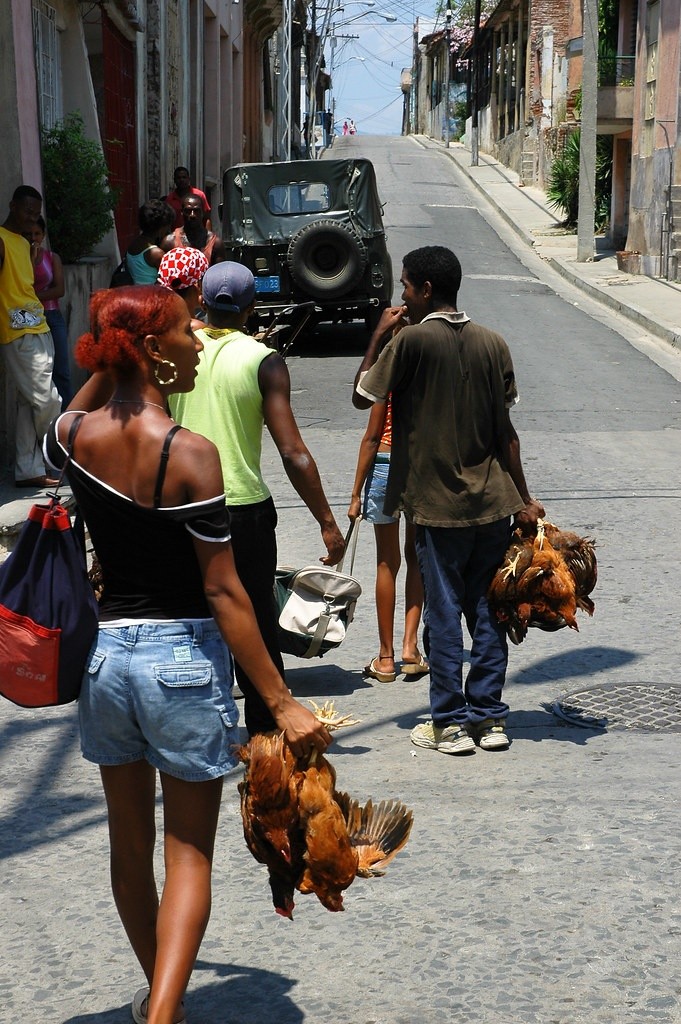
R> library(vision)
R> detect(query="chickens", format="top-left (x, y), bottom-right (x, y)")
top-left (231, 699), bottom-right (414, 922)
top-left (488, 518), bottom-right (605, 646)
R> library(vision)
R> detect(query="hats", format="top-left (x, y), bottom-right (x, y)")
top-left (155, 247), bottom-right (208, 290)
top-left (202, 261), bottom-right (255, 308)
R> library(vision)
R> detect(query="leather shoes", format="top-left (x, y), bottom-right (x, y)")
top-left (14, 476), bottom-right (63, 488)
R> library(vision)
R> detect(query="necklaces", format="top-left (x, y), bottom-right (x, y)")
top-left (109, 397), bottom-right (167, 412)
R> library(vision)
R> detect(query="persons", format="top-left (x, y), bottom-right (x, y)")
top-left (168, 260), bottom-right (346, 739)
top-left (22, 213), bottom-right (73, 414)
top-left (349, 120), bottom-right (357, 134)
top-left (0, 184), bottom-right (63, 487)
top-left (300, 116), bottom-right (314, 150)
top-left (43, 285), bottom-right (335, 1023)
top-left (161, 194), bottom-right (226, 267)
top-left (321, 109), bottom-right (327, 128)
top-left (343, 121), bottom-right (349, 136)
top-left (327, 109), bottom-right (334, 134)
top-left (165, 167), bottom-right (212, 231)
top-left (348, 317), bottom-right (430, 682)
top-left (353, 244), bottom-right (546, 756)
top-left (127, 200), bottom-right (173, 285)
top-left (157, 247), bottom-right (210, 321)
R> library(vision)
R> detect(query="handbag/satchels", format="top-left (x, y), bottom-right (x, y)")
top-left (274, 516), bottom-right (362, 660)
top-left (109, 238), bottom-right (136, 289)
top-left (0, 415), bottom-right (98, 708)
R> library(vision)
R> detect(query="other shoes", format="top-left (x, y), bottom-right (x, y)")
top-left (132, 988), bottom-right (186, 1024)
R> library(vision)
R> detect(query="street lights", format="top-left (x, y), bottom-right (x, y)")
top-left (306, 1), bottom-right (397, 156)
top-left (328, 21), bottom-right (367, 111)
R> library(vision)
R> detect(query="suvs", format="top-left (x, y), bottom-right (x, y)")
top-left (217, 157), bottom-right (394, 350)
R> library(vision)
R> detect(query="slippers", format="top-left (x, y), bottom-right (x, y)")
top-left (363, 655), bottom-right (397, 681)
top-left (401, 655), bottom-right (430, 674)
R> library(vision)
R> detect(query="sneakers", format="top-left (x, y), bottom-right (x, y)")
top-left (472, 718), bottom-right (510, 748)
top-left (410, 719), bottom-right (477, 752)
